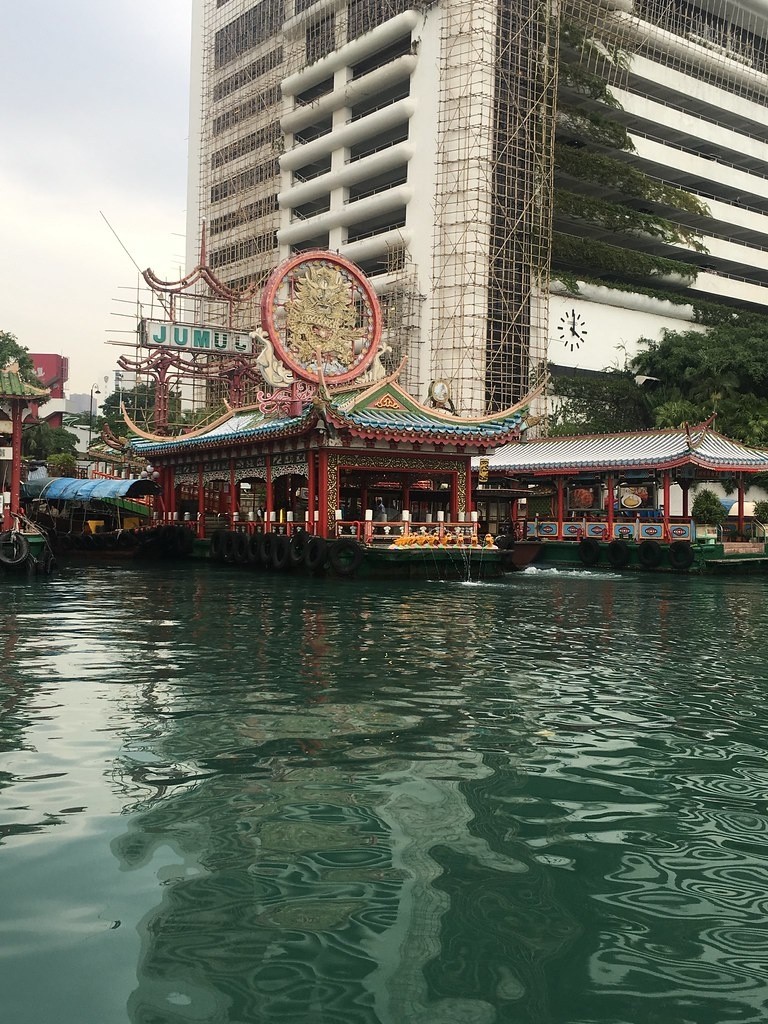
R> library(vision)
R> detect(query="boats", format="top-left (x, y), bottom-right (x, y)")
top-left (469, 412), bottom-right (767, 575)
top-left (19, 478), bottom-right (169, 557)
top-left (88, 246), bottom-right (552, 579)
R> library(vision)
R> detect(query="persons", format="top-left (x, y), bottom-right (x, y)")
top-left (372, 499), bottom-right (386, 514)
top-left (257, 505), bottom-right (264, 522)
top-left (39, 501), bottom-right (58, 517)
top-left (569, 506), bottom-right (582, 516)
